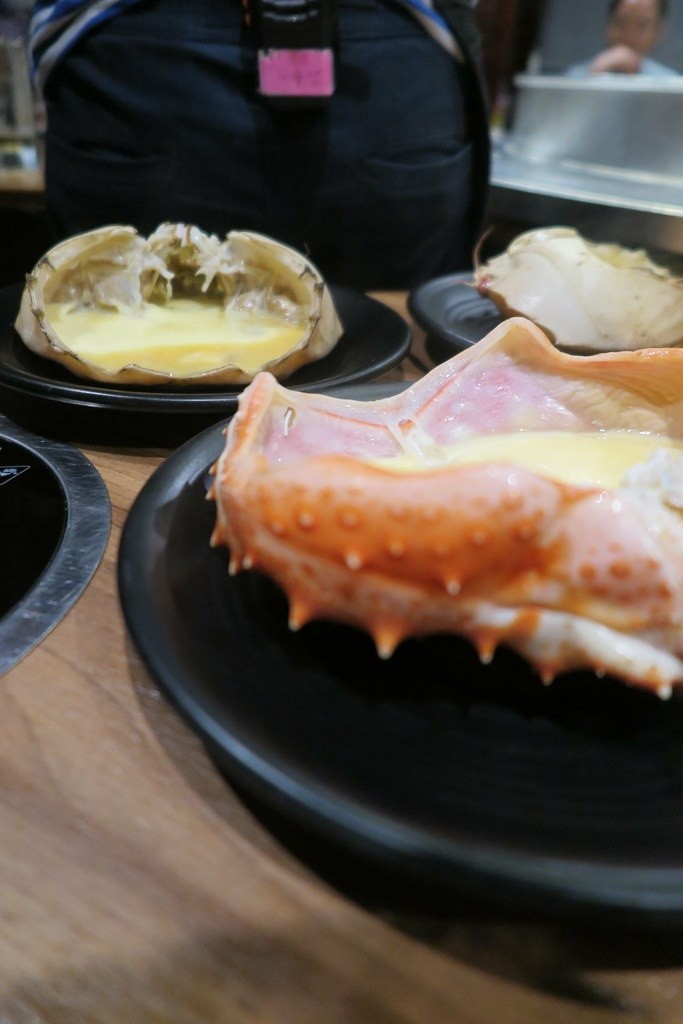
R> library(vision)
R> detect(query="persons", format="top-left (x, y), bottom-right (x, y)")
top-left (557, 0), bottom-right (681, 77)
top-left (25, 1), bottom-right (548, 293)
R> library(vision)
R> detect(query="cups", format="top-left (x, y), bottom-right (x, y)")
top-left (0, 277), bottom-right (414, 417)
top-left (0, 33), bottom-right (40, 172)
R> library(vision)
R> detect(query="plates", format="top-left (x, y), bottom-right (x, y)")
top-left (117, 381), bottom-right (683, 948)
top-left (405, 266), bottom-right (513, 351)
